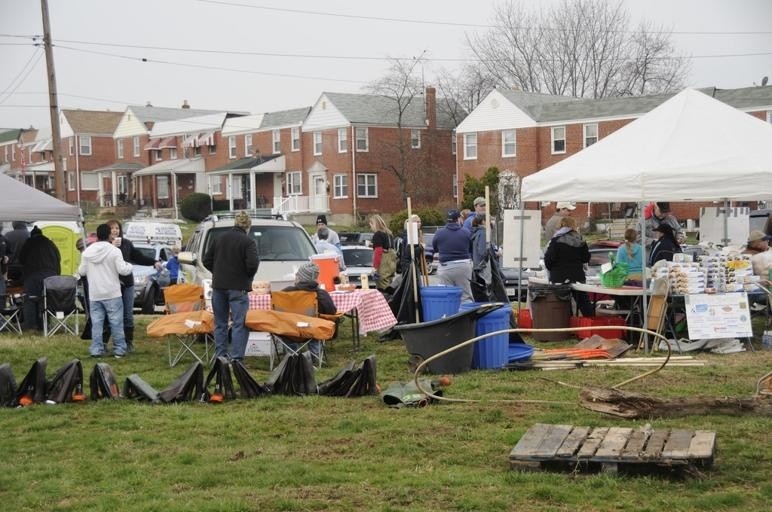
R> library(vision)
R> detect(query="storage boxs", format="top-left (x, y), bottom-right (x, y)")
top-left (245, 331), bottom-right (287, 356)
top-left (515, 308), bottom-right (627, 340)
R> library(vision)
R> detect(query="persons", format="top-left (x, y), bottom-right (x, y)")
top-left (203, 211), bottom-right (259, 371)
top-left (78, 224), bottom-right (133, 360)
top-left (370, 197), bottom-right (508, 303)
top-left (166, 246), bottom-right (182, 286)
top-left (741, 214), bottom-right (772, 326)
top-left (649, 224), bottom-right (683, 266)
top-left (281, 264), bottom-right (337, 367)
top-left (311, 215), bottom-right (341, 251)
top-left (615, 229), bottom-right (642, 286)
top-left (636, 202), bottom-right (687, 266)
top-left (4, 221), bottom-right (31, 323)
top-left (544, 218), bottom-right (594, 317)
top-left (19, 228), bottom-right (61, 335)
top-left (314, 228), bottom-right (346, 271)
top-left (102, 220), bottom-right (164, 353)
top-left (545, 202), bottom-right (577, 245)
top-left (0, 221), bottom-right (14, 331)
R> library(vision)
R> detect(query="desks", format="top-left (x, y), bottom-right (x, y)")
top-left (527, 274), bottom-right (657, 347)
top-left (210, 284), bottom-right (399, 357)
top-left (667, 288), bottom-right (772, 353)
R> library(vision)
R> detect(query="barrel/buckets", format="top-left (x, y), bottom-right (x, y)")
top-left (516, 308), bottom-right (532, 336)
top-left (308, 254), bottom-right (343, 292)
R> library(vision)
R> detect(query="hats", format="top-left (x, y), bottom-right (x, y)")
top-left (656, 202), bottom-right (671, 213)
top-left (460, 209), bottom-right (471, 217)
top-left (447, 208), bottom-right (460, 220)
top-left (294, 262), bottom-right (319, 284)
top-left (652, 223), bottom-right (673, 233)
top-left (30, 225), bottom-right (42, 235)
top-left (556, 201), bottom-right (577, 210)
top-left (234, 212), bottom-right (252, 229)
top-left (473, 196), bottom-right (486, 206)
top-left (316, 215), bottom-right (326, 225)
top-left (747, 230), bottom-right (772, 242)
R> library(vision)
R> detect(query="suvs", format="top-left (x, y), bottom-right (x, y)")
top-left (176, 213), bottom-right (319, 344)
top-left (422, 233), bottom-right (437, 274)
top-left (71, 242), bottom-right (176, 309)
top-left (338, 230), bottom-right (375, 246)
top-left (747, 210), bottom-right (769, 317)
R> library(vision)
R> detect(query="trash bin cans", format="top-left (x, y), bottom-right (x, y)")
top-left (311, 254), bottom-right (340, 292)
top-left (584, 218), bottom-right (591, 232)
top-left (528, 285), bottom-right (571, 342)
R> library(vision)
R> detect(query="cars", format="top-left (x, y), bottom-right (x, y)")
top-left (341, 246), bottom-right (379, 290)
top-left (335, 242), bottom-right (366, 249)
top-left (524, 248), bottom-right (562, 302)
top-left (495, 247), bottom-right (530, 303)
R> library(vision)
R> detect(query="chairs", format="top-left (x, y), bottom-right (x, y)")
top-left (636, 276), bottom-right (682, 355)
top-left (265, 236), bottom-right (298, 261)
top-left (0, 280), bottom-right (23, 340)
top-left (39, 272), bottom-right (83, 338)
top-left (270, 291), bottom-right (331, 369)
top-left (163, 282), bottom-right (209, 367)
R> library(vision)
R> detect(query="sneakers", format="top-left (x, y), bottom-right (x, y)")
top-left (115, 355), bottom-right (124, 359)
top-left (89, 353), bottom-right (103, 356)
